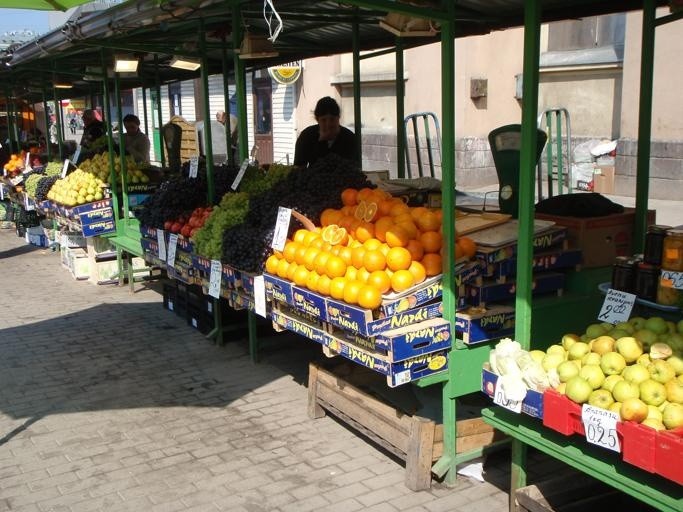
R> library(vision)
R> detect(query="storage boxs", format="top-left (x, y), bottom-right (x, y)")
top-left (533, 207), bottom-right (656, 269)
top-left (25, 219), bottom-right (160, 284)
top-left (593, 165), bottom-right (615, 194)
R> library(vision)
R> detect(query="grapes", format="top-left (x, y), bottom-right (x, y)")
top-left (22, 147), bottom-right (105, 198)
top-left (132, 153), bottom-right (377, 273)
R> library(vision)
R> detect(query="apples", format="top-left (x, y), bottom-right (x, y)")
top-left (529, 317), bottom-right (683, 432)
top-left (46, 150), bottom-right (149, 207)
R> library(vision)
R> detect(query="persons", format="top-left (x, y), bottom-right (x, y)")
top-left (80, 109), bottom-right (106, 148)
top-left (123, 113), bottom-right (150, 163)
top-left (69, 118), bottom-right (77, 134)
top-left (216, 110), bottom-right (241, 166)
top-left (293, 96), bottom-right (356, 169)
top-left (49, 113), bottom-right (57, 122)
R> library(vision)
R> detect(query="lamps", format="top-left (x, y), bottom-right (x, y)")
top-left (113, 54), bottom-right (139, 74)
top-left (379, 9), bottom-right (436, 38)
top-left (170, 55), bottom-right (201, 71)
top-left (238, 30), bottom-right (279, 59)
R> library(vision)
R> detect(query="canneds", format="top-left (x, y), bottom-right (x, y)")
top-left (612, 225), bottom-right (682, 306)
